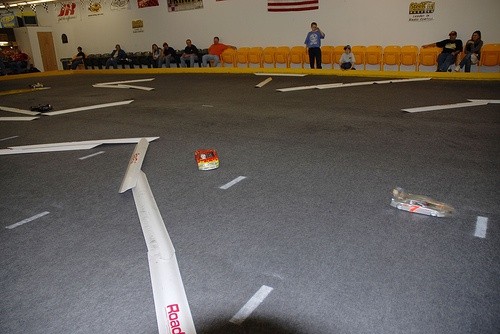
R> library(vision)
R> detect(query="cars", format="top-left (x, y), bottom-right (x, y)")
top-left (30, 104), bottom-right (51, 112)
top-left (29, 83), bottom-right (42, 88)
top-left (195, 147), bottom-right (220, 170)
top-left (390, 186), bottom-right (457, 217)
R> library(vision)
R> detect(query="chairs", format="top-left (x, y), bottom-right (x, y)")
top-left (83, 44), bottom-right (500, 71)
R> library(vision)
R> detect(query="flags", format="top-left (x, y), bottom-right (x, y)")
top-left (267, 0), bottom-right (320, 13)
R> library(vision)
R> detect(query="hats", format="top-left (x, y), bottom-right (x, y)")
top-left (344, 45), bottom-right (351, 50)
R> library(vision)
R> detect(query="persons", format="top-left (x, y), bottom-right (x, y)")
top-left (180, 39), bottom-right (198, 68)
top-left (72, 47), bottom-right (85, 70)
top-left (202, 37), bottom-right (227, 68)
top-left (148, 44), bottom-right (165, 68)
top-left (304, 22), bottom-right (325, 69)
top-left (158, 43), bottom-right (176, 68)
top-left (105, 44), bottom-right (125, 69)
top-left (454, 31), bottom-right (483, 73)
top-left (422, 31), bottom-right (463, 72)
top-left (339, 45), bottom-right (355, 70)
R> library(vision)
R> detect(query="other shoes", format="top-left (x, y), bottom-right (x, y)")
top-left (455, 66), bottom-right (460, 71)
